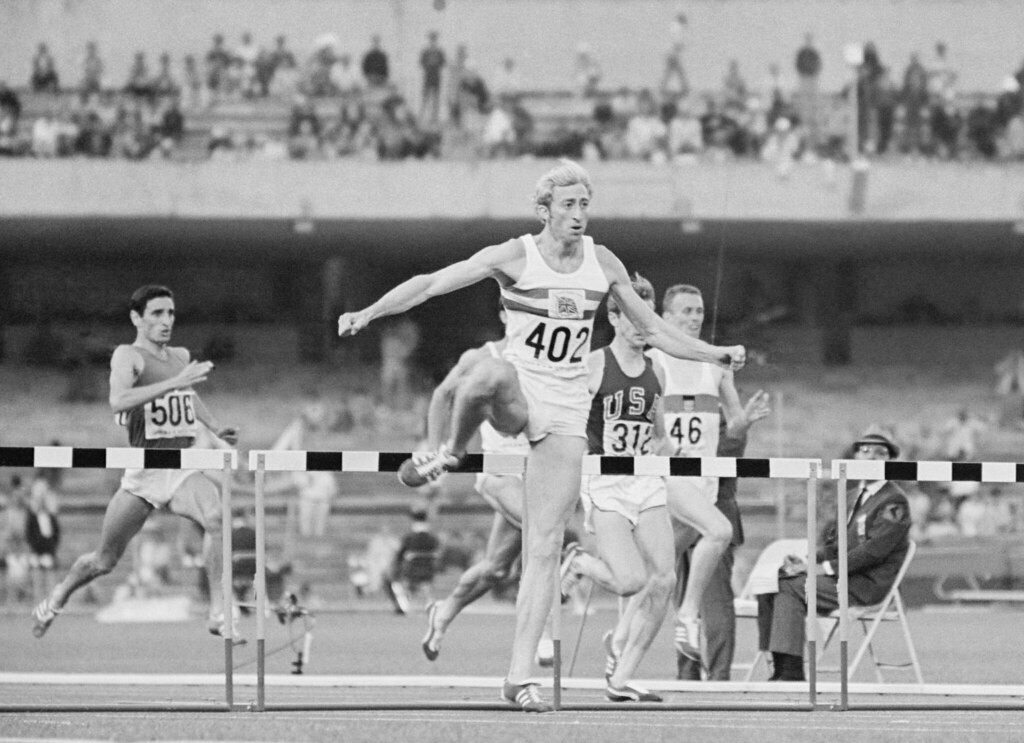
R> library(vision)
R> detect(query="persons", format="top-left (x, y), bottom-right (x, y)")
top-left (0, 162), bottom-right (1024, 712)
top-left (0, 11), bottom-right (1024, 167)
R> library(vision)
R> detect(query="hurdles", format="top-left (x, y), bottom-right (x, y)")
top-left (0, 444), bottom-right (1024, 709)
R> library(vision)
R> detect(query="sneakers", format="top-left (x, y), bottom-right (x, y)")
top-left (32, 596), bottom-right (60, 638)
top-left (398, 445), bottom-right (466, 487)
top-left (503, 677), bottom-right (551, 712)
top-left (674, 607), bottom-right (702, 662)
top-left (603, 630), bottom-right (621, 684)
top-left (423, 599), bottom-right (447, 662)
top-left (208, 613), bottom-right (248, 646)
top-left (560, 542), bottom-right (587, 603)
top-left (605, 676), bottom-right (664, 703)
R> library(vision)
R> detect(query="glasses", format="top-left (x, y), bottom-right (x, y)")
top-left (857, 445), bottom-right (889, 457)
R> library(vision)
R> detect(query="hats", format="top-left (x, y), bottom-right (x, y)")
top-left (851, 425), bottom-right (901, 460)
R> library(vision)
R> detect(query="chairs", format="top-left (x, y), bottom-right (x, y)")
top-left (817, 539), bottom-right (923, 684)
top-left (701, 537), bottom-right (809, 680)
